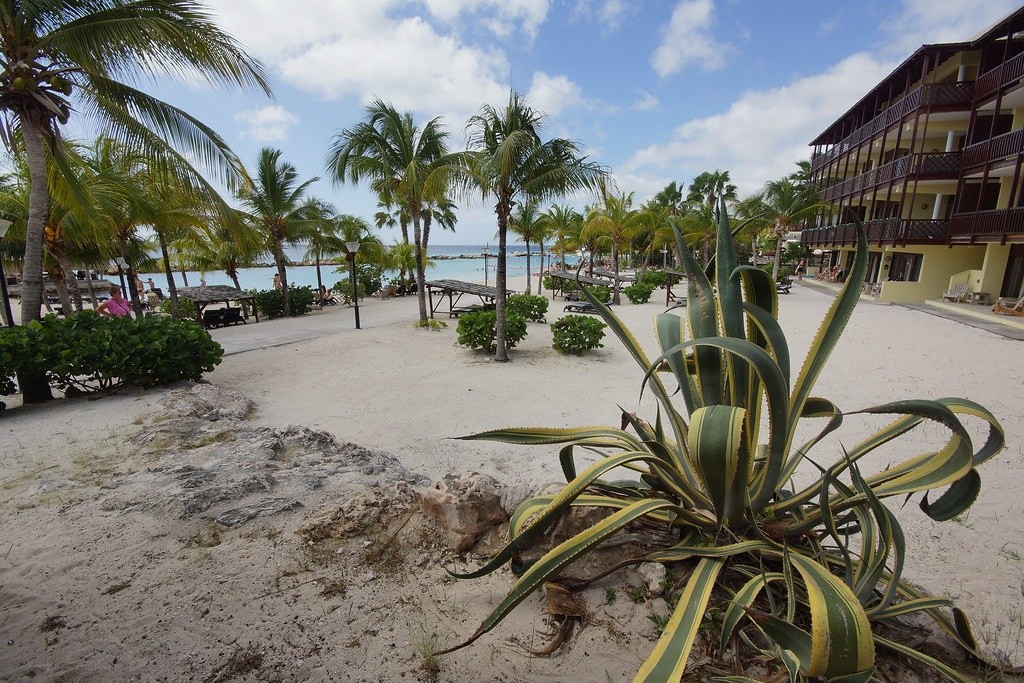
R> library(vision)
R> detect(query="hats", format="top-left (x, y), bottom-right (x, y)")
top-left (274, 273), bottom-right (279, 276)
top-left (138, 288), bottom-right (142, 292)
top-left (148, 278), bottom-right (152, 280)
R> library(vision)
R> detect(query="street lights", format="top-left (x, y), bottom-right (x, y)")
top-left (481, 247), bottom-right (489, 286)
top-left (0, 219), bottom-right (15, 326)
top-left (114, 254), bottom-right (129, 301)
top-left (344, 241), bottom-right (361, 330)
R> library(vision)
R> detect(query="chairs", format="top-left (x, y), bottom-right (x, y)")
top-left (147, 293), bottom-right (161, 310)
top-left (942, 283), bottom-right (970, 304)
top-left (312, 296), bottom-right (338, 306)
top-left (562, 303), bottom-right (614, 314)
top-left (775, 284), bottom-right (792, 294)
top-left (993, 295), bottom-right (1024, 316)
top-left (203, 308), bottom-right (246, 329)
top-left (375, 283), bottom-right (418, 300)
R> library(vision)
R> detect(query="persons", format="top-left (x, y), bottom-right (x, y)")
top-left (272, 274), bottom-right (331, 306)
top-left (888, 272), bottom-right (904, 281)
top-left (795, 261), bottom-right (842, 284)
top-left (550, 258), bottom-right (611, 272)
top-left (382, 275), bottom-right (416, 296)
top-left (96, 278), bottom-right (155, 318)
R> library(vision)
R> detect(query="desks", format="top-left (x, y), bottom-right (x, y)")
top-left (781, 279), bottom-right (793, 286)
top-left (966, 292), bottom-right (990, 306)
